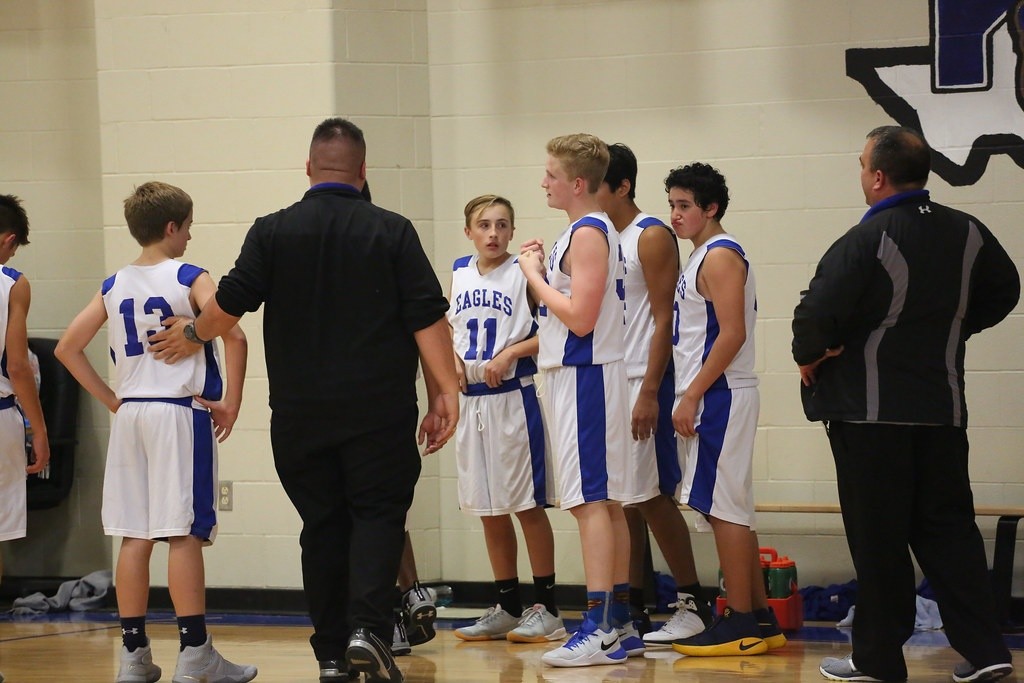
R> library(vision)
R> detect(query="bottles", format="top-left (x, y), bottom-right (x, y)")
top-left (718, 556), bottom-right (798, 598)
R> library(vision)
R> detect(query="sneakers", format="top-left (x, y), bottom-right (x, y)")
top-left (752, 608), bottom-right (789, 648)
top-left (507, 603), bottom-right (568, 644)
top-left (540, 610), bottom-right (629, 668)
top-left (391, 609), bottom-right (418, 656)
top-left (669, 608), bottom-right (767, 659)
top-left (638, 592), bottom-right (717, 646)
top-left (819, 651), bottom-right (898, 682)
top-left (629, 609), bottom-right (657, 638)
top-left (345, 625), bottom-right (405, 683)
top-left (171, 632), bottom-right (258, 683)
top-left (317, 655), bottom-right (363, 683)
top-left (453, 602), bottom-right (523, 640)
top-left (612, 607), bottom-right (645, 658)
top-left (948, 658), bottom-right (1015, 683)
top-left (113, 640), bottom-right (162, 683)
top-left (402, 579), bottom-right (438, 646)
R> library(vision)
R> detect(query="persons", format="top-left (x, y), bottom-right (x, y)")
top-left (54, 182), bottom-right (260, 683)
top-left (792, 126), bottom-right (1021, 683)
top-left (665, 163), bottom-right (786, 655)
top-left (596, 142), bottom-right (716, 643)
top-left (0, 195), bottom-right (49, 542)
top-left (144, 118), bottom-right (463, 682)
top-left (446, 194), bottom-right (568, 642)
top-left (516, 134), bottom-right (648, 666)
top-left (390, 351), bottom-right (440, 657)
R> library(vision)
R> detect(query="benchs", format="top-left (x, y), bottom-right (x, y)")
top-left (675, 495), bottom-right (1024, 605)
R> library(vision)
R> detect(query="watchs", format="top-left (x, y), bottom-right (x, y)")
top-left (183, 320), bottom-right (210, 344)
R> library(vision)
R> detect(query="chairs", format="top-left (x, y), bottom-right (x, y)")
top-left (1, 336), bottom-right (78, 507)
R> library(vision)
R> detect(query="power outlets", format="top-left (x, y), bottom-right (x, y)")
top-left (217, 481), bottom-right (234, 512)
